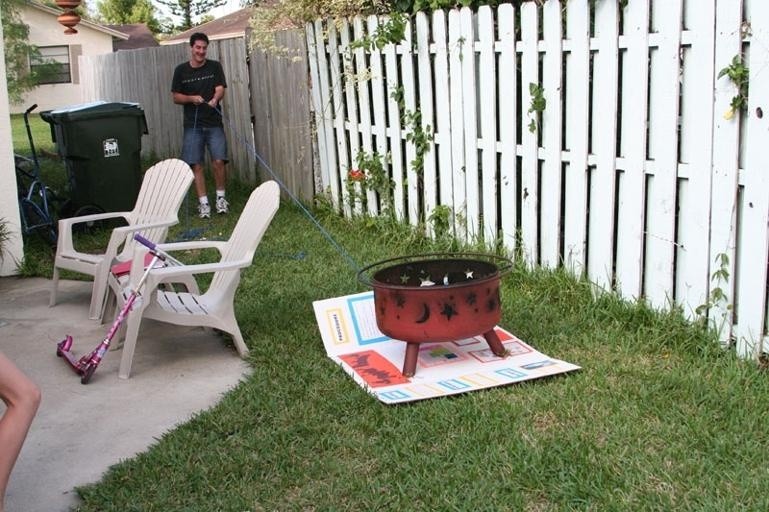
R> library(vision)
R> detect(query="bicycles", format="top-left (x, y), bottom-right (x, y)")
top-left (15, 103), bottom-right (56, 254)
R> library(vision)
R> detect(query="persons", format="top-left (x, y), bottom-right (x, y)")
top-left (171, 33), bottom-right (229, 220)
top-left (0, 354), bottom-right (41, 512)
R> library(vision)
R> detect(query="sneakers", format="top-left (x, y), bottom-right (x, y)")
top-left (215, 197), bottom-right (229, 215)
top-left (198, 203), bottom-right (212, 218)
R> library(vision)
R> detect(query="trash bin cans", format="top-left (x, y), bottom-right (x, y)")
top-left (39, 100), bottom-right (149, 237)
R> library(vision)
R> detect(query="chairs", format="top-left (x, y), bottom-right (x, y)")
top-left (50, 156), bottom-right (197, 320)
top-left (112, 179), bottom-right (283, 382)
